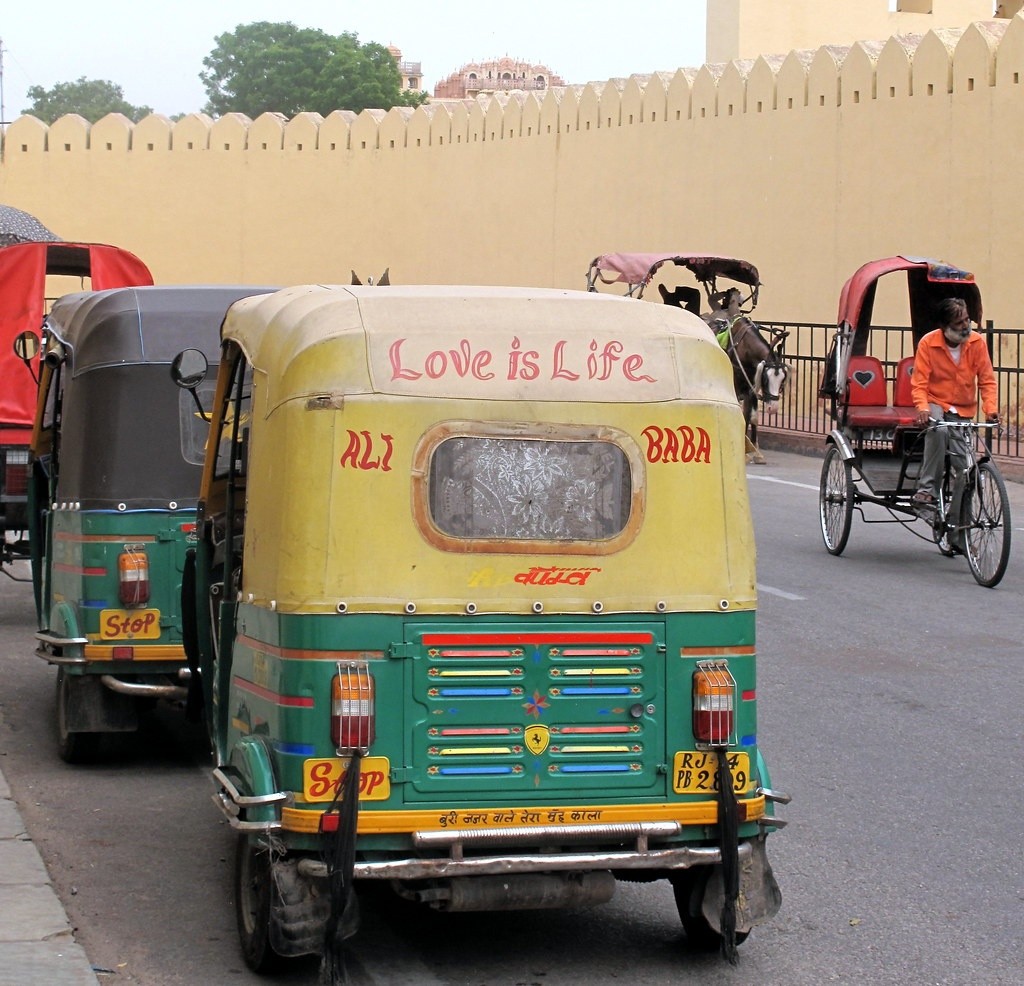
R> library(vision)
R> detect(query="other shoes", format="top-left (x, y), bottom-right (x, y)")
top-left (970, 545), bottom-right (978, 556)
top-left (913, 491), bottom-right (932, 503)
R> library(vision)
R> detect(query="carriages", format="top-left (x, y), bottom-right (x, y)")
top-left (586, 252), bottom-right (792, 464)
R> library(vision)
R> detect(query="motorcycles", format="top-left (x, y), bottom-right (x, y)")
top-left (11, 283), bottom-right (285, 769)
top-left (165, 286), bottom-right (794, 986)
top-left (0, 204), bottom-right (158, 564)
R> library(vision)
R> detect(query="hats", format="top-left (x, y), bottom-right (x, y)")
top-left (738, 295), bottom-right (744, 306)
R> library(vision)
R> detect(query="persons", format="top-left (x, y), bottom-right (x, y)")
top-left (909, 297), bottom-right (1001, 562)
top-left (659, 281), bottom-right (741, 330)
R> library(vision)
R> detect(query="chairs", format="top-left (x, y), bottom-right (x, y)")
top-left (891, 356), bottom-right (924, 427)
top-left (838, 355), bottom-right (898, 426)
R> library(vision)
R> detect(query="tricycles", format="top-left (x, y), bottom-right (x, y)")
top-left (815, 255), bottom-right (1012, 589)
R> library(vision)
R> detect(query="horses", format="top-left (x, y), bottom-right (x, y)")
top-left (707, 316), bottom-right (790, 465)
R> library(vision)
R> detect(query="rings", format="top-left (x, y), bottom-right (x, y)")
top-left (917, 420), bottom-right (921, 422)
